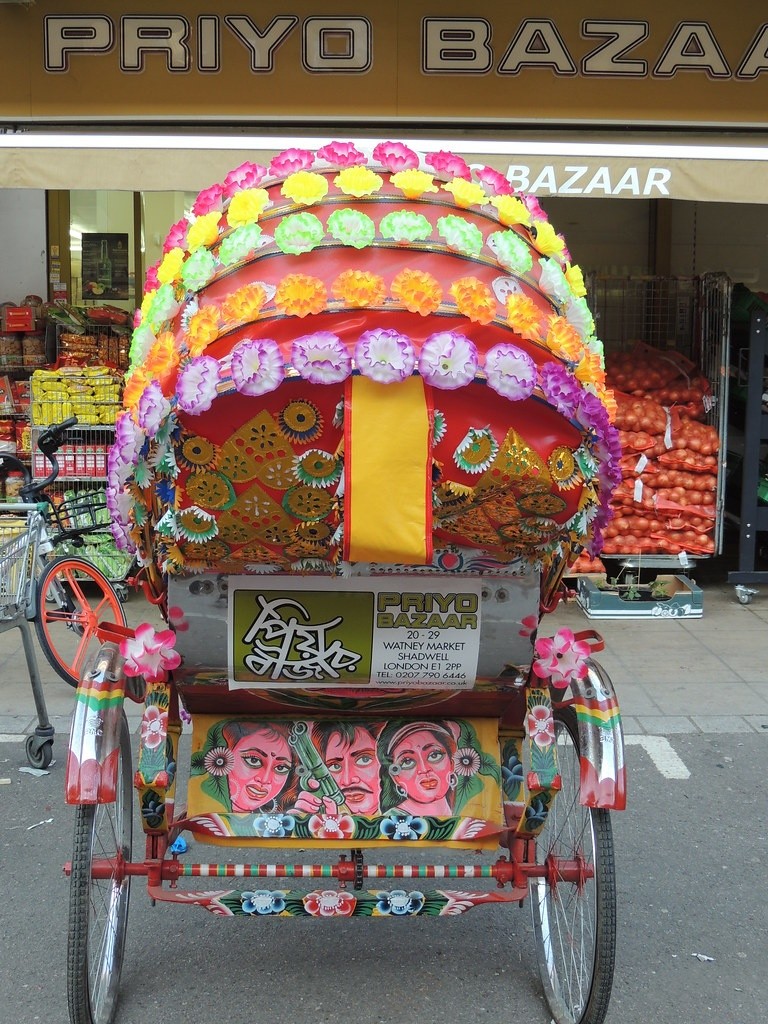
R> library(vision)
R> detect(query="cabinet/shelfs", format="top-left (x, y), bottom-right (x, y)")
top-left (716, 306), bottom-right (768, 584)
top-left (0, 323), bottom-right (138, 581)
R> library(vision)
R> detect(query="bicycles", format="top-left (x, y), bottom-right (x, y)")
top-left (1, 417), bottom-right (127, 689)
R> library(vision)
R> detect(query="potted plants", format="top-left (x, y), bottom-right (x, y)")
top-left (594, 576), bottom-right (672, 601)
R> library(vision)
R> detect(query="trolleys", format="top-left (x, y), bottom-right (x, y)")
top-left (0, 504), bottom-right (56, 768)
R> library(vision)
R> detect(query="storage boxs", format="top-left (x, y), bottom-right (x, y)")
top-left (577, 575), bottom-right (704, 620)
top-left (0, 305), bottom-right (35, 332)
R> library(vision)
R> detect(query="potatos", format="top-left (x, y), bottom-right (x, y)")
top-left (565, 343), bottom-right (721, 573)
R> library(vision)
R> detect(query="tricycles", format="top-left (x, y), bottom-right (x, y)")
top-left (61, 140), bottom-right (627, 1024)
